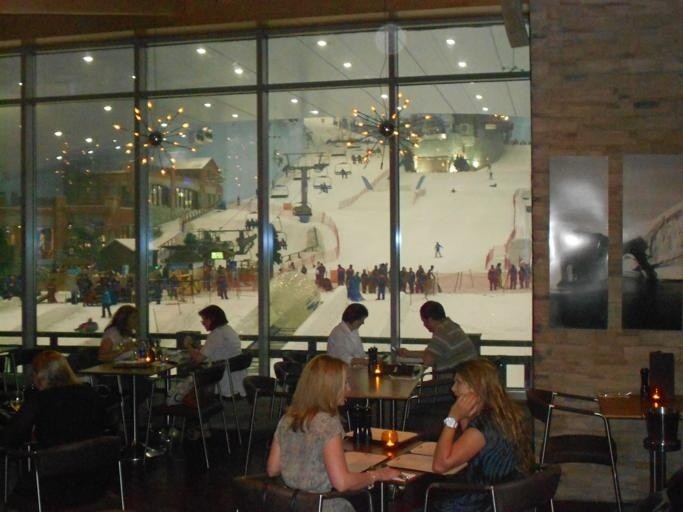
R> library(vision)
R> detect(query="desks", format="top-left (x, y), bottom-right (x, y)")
top-left (600, 394), bottom-right (683, 492)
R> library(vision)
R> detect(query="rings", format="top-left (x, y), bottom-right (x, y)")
top-left (461, 396), bottom-right (467, 400)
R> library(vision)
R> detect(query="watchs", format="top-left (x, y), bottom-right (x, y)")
top-left (443, 418), bottom-right (459, 428)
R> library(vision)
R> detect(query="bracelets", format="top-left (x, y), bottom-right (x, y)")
top-left (367, 469), bottom-right (376, 490)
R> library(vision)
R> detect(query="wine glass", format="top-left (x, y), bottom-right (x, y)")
top-left (10, 391), bottom-right (24, 413)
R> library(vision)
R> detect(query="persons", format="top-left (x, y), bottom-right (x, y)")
top-left (400, 264), bottom-right (436, 293)
top-left (325, 302), bottom-right (383, 368)
top-left (1, 260), bottom-right (139, 318)
top-left (433, 241), bottom-right (444, 258)
top-left (148, 260), bottom-right (258, 304)
top-left (431, 356), bottom-right (536, 511)
top-left (273, 260), bottom-right (390, 300)
top-left (488, 262), bottom-right (532, 291)
top-left (421, 299), bottom-right (478, 375)
top-left (190, 304), bottom-right (248, 398)
top-left (265, 352), bottom-right (402, 512)
top-left (6, 347), bottom-right (106, 509)
top-left (96, 306), bottom-right (139, 363)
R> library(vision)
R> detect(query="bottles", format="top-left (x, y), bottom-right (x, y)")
top-left (351, 404), bottom-right (371, 441)
top-left (367, 346), bottom-right (377, 373)
top-left (131, 329), bottom-right (167, 363)
top-left (639, 367), bottom-right (650, 397)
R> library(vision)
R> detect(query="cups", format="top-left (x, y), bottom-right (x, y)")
top-left (373, 361), bottom-right (382, 375)
top-left (381, 431), bottom-right (398, 450)
top-left (650, 385), bottom-right (662, 401)
